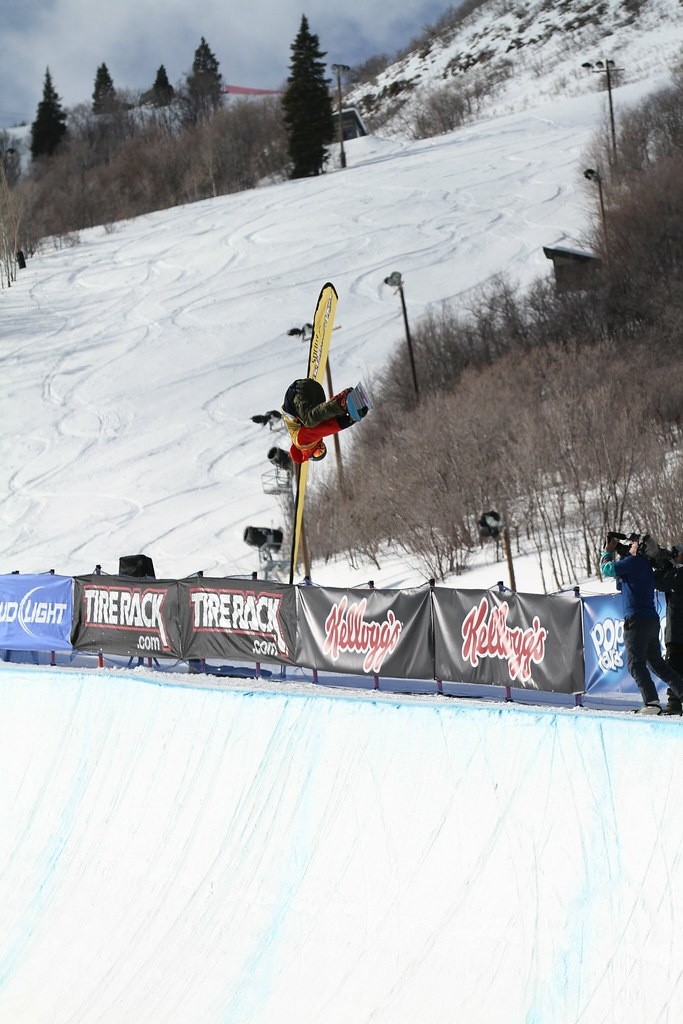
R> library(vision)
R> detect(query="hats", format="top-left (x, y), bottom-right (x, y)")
top-left (309, 442), bottom-right (327, 461)
top-left (638, 534), bottom-right (658, 557)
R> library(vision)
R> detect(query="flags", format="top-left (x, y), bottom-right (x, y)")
top-left (290, 282), bottom-right (338, 576)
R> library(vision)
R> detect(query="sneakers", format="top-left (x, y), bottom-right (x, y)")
top-left (662, 704), bottom-right (682, 713)
top-left (640, 700), bottom-right (663, 715)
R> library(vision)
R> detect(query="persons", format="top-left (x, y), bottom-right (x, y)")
top-left (280, 378), bottom-right (368, 465)
top-left (600, 533), bottom-right (683, 716)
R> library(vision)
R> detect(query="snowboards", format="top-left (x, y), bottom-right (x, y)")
top-left (347, 382), bottom-right (375, 421)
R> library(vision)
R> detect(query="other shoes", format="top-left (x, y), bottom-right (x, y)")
top-left (338, 387), bottom-right (353, 411)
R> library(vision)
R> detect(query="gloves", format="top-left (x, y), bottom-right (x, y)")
top-left (605, 536), bottom-right (619, 553)
top-left (357, 406), bottom-right (368, 418)
